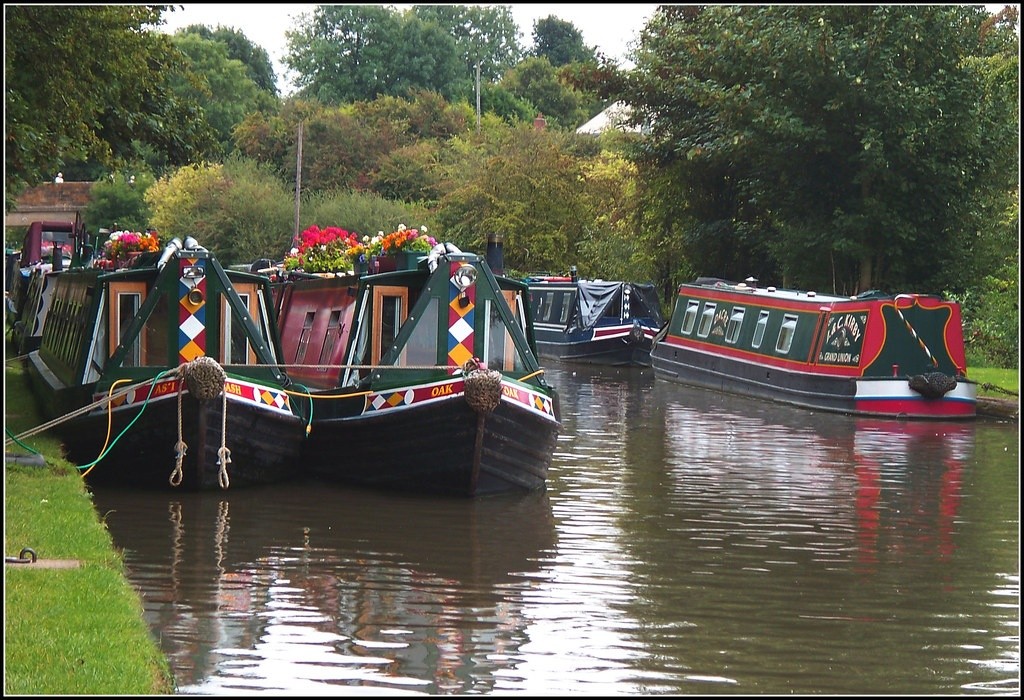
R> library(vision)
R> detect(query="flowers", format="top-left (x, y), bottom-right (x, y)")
top-left (101, 230), bottom-right (159, 260)
top-left (284, 225), bottom-right (439, 274)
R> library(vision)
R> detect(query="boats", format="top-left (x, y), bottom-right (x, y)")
top-left (27, 211), bottom-right (307, 489)
top-left (5, 220), bottom-right (78, 354)
top-left (522, 265), bottom-right (667, 366)
top-left (224, 233), bottom-right (560, 503)
top-left (629, 316), bottom-right (660, 365)
top-left (650, 277), bottom-right (980, 424)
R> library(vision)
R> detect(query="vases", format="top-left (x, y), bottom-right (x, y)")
top-left (126, 251), bottom-right (142, 265)
top-left (370, 256), bottom-right (397, 272)
top-left (112, 259), bottom-right (123, 270)
top-left (354, 260), bottom-right (368, 274)
top-left (394, 251), bottom-right (427, 269)
